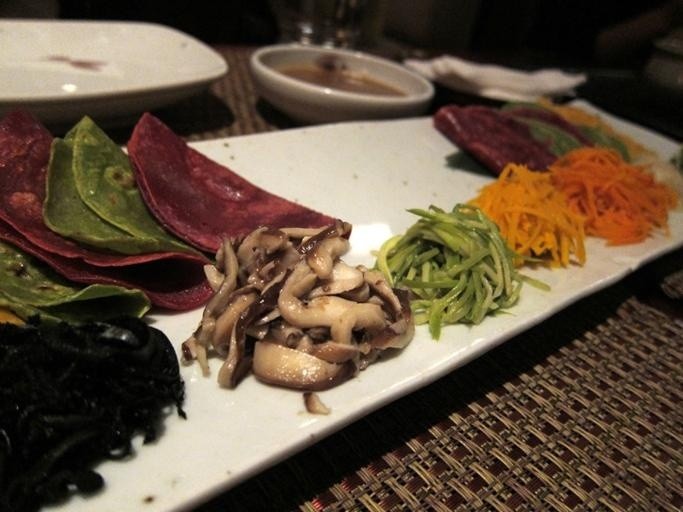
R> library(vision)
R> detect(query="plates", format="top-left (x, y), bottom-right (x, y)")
top-left (35, 93), bottom-right (683, 512)
top-left (248, 43), bottom-right (436, 124)
top-left (1, 18), bottom-right (230, 126)
